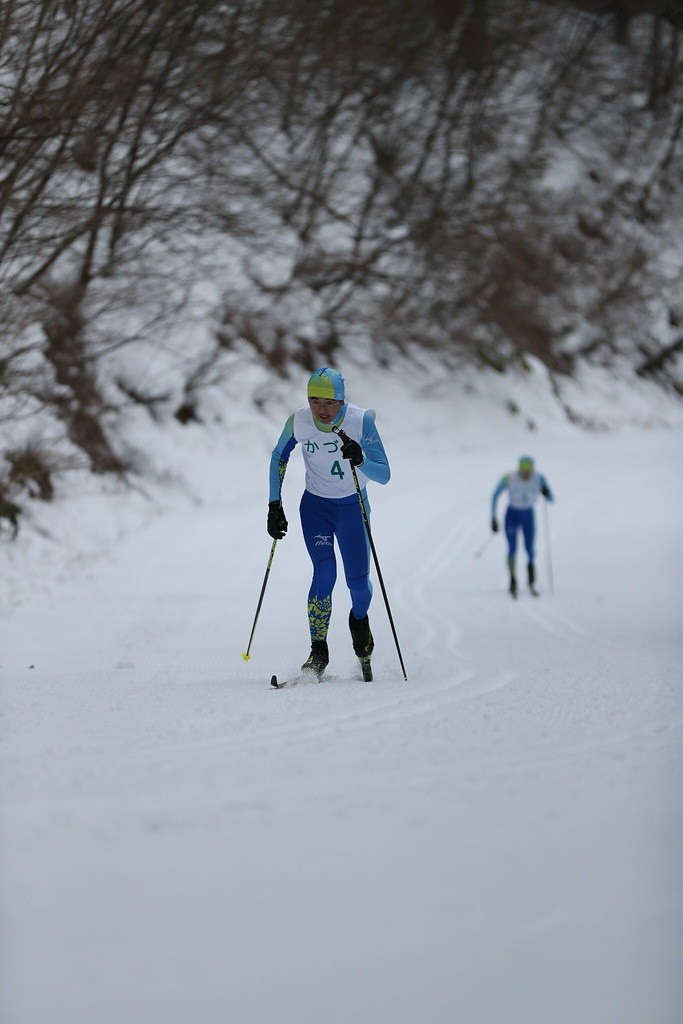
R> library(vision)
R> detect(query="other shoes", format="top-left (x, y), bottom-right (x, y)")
top-left (528, 584), bottom-right (539, 596)
top-left (509, 578), bottom-right (517, 593)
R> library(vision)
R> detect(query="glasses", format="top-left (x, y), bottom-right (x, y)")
top-left (308, 399), bottom-right (343, 407)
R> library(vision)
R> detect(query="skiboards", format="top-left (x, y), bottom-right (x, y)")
top-left (269, 659), bottom-right (374, 689)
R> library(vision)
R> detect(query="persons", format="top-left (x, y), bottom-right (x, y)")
top-left (492, 457), bottom-right (553, 593)
top-left (267, 367), bottom-right (391, 675)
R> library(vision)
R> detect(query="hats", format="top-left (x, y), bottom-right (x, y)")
top-left (520, 456), bottom-right (534, 472)
top-left (307, 368), bottom-right (346, 400)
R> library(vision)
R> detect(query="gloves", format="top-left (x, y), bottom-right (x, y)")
top-left (266, 501), bottom-right (288, 540)
top-left (492, 519), bottom-right (499, 533)
top-left (540, 484), bottom-right (550, 497)
top-left (338, 430), bottom-right (364, 466)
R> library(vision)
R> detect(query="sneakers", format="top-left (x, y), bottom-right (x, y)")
top-left (348, 609), bottom-right (375, 658)
top-left (300, 641), bottom-right (329, 675)
